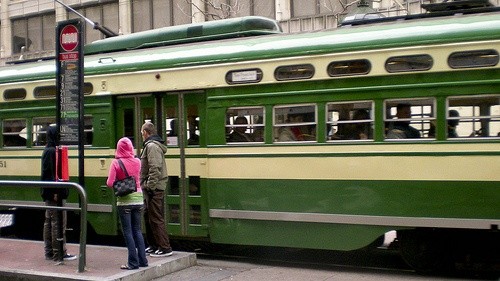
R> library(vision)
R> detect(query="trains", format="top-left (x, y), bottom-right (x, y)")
top-left (1, 0), bottom-right (499, 254)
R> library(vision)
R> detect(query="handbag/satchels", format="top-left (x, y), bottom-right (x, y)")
top-left (112, 159), bottom-right (137, 198)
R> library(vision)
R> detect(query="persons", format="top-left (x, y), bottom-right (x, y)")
top-left (106, 137), bottom-right (148, 269)
top-left (3, 103), bottom-right (477, 146)
top-left (40, 126), bottom-right (77, 261)
top-left (139, 122), bottom-right (173, 257)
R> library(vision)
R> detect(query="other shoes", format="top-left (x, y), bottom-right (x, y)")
top-left (120, 263), bottom-right (134, 269)
top-left (46, 256), bottom-right (53, 259)
top-left (150, 249), bottom-right (173, 257)
top-left (63, 254), bottom-right (77, 260)
top-left (145, 246), bottom-right (154, 253)
